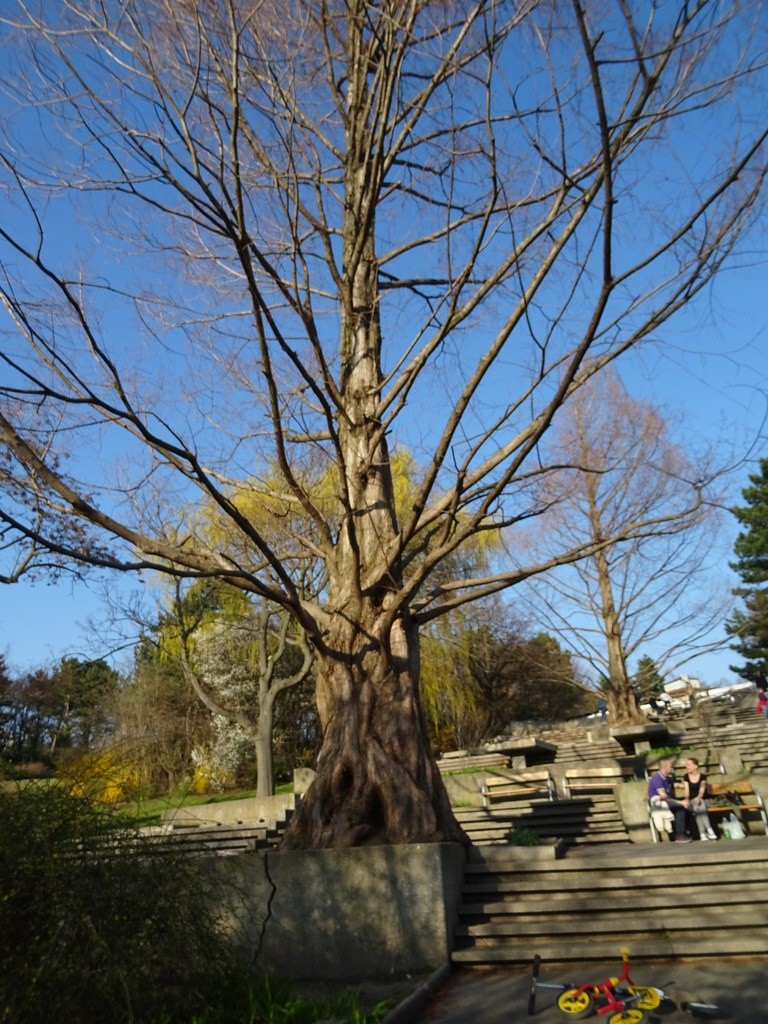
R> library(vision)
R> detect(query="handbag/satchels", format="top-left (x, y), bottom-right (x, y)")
top-left (723, 813), bottom-right (746, 840)
top-left (728, 790), bottom-right (745, 806)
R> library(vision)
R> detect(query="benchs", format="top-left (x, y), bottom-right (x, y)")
top-left (644, 781), bottom-right (768, 843)
top-left (643, 757), bottom-right (725, 781)
top-left (562, 768), bottom-right (635, 799)
top-left (482, 771), bottom-right (553, 806)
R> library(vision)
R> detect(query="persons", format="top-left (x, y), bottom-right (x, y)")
top-left (649, 693), bottom-right (672, 715)
top-left (648, 758), bottom-right (718, 844)
top-left (751, 667), bottom-right (768, 721)
top-left (598, 698), bottom-right (608, 722)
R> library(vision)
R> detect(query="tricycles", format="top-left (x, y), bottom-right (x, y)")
top-left (555, 943), bottom-right (663, 1024)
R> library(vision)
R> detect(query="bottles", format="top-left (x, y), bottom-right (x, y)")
top-left (722, 817), bottom-right (731, 840)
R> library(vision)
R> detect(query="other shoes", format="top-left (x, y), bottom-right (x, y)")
top-left (707, 828), bottom-right (717, 839)
top-left (675, 836), bottom-right (692, 843)
top-left (700, 833), bottom-right (709, 840)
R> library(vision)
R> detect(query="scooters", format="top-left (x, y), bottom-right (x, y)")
top-left (528, 944), bottom-right (729, 1024)
